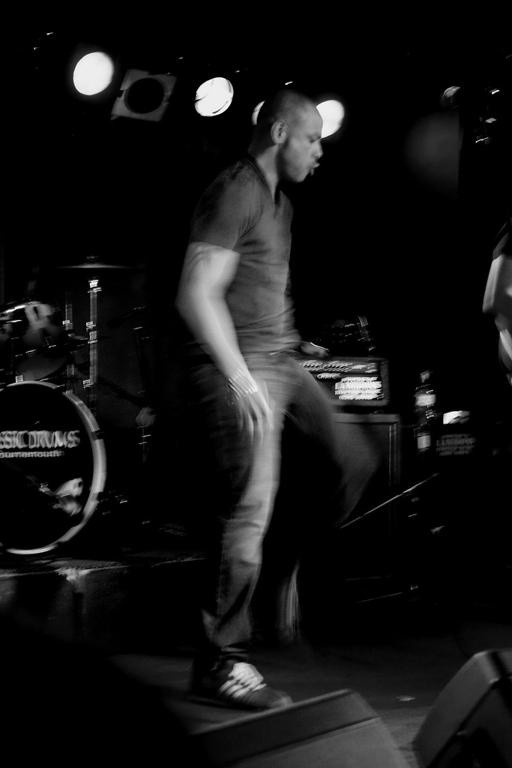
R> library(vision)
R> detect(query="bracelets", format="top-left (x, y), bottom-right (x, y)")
top-left (224, 366), bottom-right (262, 404)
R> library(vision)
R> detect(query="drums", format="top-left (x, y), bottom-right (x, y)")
top-left (1, 381), bottom-right (107, 554)
top-left (1, 301), bottom-right (69, 381)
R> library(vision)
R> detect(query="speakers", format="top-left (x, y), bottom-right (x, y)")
top-left (412, 647), bottom-right (512, 768)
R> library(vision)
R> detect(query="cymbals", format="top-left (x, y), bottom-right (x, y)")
top-left (64, 260), bottom-right (129, 270)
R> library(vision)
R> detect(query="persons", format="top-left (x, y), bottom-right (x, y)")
top-left (171, 91), bottom-right (377, 712)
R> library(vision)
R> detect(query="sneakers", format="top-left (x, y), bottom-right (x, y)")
top-left (191, 655), bottom-right (292, 716)
